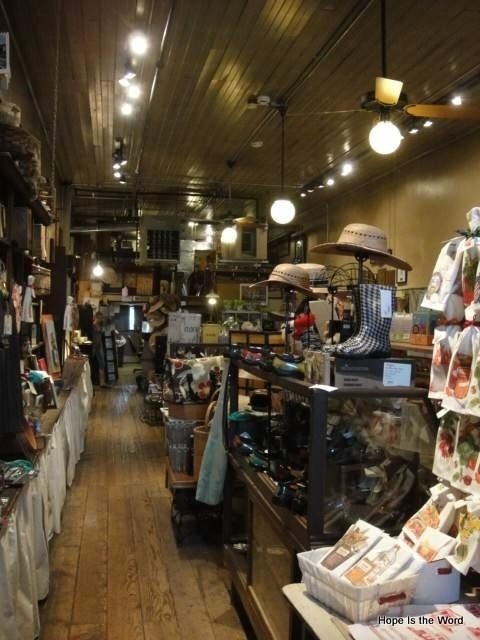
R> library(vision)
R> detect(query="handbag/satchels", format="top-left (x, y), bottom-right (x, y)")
top-left (192, 402), bottom-right (217, 482)
top-left (195, 358), bottom-right (230, 505)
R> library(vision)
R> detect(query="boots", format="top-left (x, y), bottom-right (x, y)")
top-left (343, 284), bottom-right (398, 358)
top-left (318, 285), bottom-right (368, 353)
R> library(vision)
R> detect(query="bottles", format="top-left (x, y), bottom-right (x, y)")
top-left (363, 545), bottom-right (399, 585)
top-left (71, 330), bottom-right (88, 345)
top-left (230, 344), bottom-right (302, 375)
top-left (218, 325), bottom-right (229, 345)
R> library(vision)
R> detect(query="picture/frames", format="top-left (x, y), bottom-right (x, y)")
top-left (39, 314), bottom-right (62, 379)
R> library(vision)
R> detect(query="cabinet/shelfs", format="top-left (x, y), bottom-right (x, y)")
top-left (223, 351), bottom-right (439, 640)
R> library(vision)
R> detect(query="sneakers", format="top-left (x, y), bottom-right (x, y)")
top-left (225, 389), bottom-right (418, 514)
top-left (223, 340), bottom-right (308, 380)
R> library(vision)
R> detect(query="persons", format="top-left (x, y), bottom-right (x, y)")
top-left (179, 270), bottom-right (205, 307)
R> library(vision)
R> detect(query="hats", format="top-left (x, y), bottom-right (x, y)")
top-left (313, 221), bottom-right (412, 269)
top-left (296, 261), bottom-right (333, 294)
top-left (250, 263), bottom-right (317, 296)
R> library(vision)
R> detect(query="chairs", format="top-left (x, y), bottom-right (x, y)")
top-left (127, 334), bottom-right (143, 373)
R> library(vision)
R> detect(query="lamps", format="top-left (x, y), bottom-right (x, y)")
top-left (269, 107), bottom-right (296, 225)
top-left (369, 34), bottom-right (403, 156)
top-left (112, 148), bottom-right (128, 185)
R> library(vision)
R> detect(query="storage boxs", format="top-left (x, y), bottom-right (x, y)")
top-left (168, 311), bottom-right (202, 344)
top-left (201, 324), bottom-right (219, 344)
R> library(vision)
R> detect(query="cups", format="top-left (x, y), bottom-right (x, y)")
top-left (455, 376), bottom-right (470, 399)
top-left (321, 544), bottom-right (359, 570)
top-left (345, 556), bottom-right (378, 585)
top-left (389, 313), bottom-right (412, 343)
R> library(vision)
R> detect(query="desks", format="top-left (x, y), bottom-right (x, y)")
top-left (163, 454), bottom-right (231, 548)
top-left (283, 583), bottom-right (480, 640)
top-left (0, 352), bottom-right (96, 640)
top-left (159, 406), bottom-right (208, 450)
